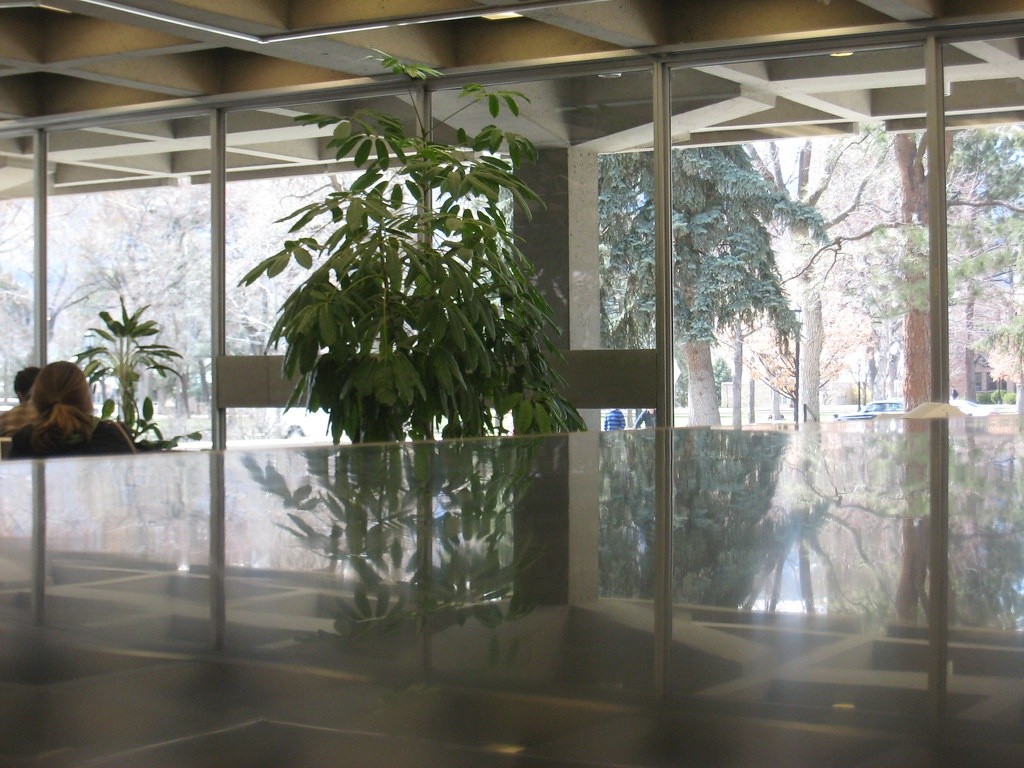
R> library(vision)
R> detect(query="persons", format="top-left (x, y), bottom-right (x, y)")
top-left (632, 409), bottom-right (655, 429)
top-left (951, 386), bottom-right (958, 401)
top-left (600, 409), bottom-right (626, 432)
top-left (0, 360), bottom-right (138, 460)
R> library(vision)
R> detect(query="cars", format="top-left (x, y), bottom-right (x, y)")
top-left (843, 401), bottom-right (906, 420)
